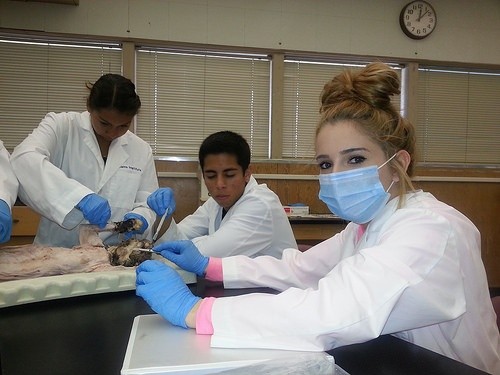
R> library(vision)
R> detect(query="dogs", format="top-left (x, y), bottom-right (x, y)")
top-left (0, 218), bottom-right (155, 281)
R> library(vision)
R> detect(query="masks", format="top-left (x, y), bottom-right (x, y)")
top-left (319, 153), bottom-right (398, 225)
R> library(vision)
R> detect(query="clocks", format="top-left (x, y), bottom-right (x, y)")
top-left (399, 0), bottom-right (438, 40)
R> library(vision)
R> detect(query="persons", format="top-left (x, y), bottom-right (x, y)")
top-left (0, 137), bottom-right (20, 245)
top-left (146, 129), bottom-right (300, 261)
top-left (10, 73), bottom-right (160, 247)
top-left (135, 59), bottom-right (500, 375)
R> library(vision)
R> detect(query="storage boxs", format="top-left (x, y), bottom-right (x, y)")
top-left (283, 205), bottom-right (310, 215)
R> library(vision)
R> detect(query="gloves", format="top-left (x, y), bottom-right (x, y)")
top-left (152, 240), bottom-right (209, 277)
top-left (76, 192), bottom-right (111, 229)
top-left (123, 212), bottom-right (149, 241)
top-left (135, 260), bottom-right (202, 329)
top-left (0, 198), bottom-right (13, 244)
top-left (147, 187), bottom-right (176, 218)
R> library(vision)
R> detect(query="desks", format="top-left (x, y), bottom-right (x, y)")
top-left (289, 212), bottom-right (348, 241)
top-left (0, 276), bottom-right (491, 375)
